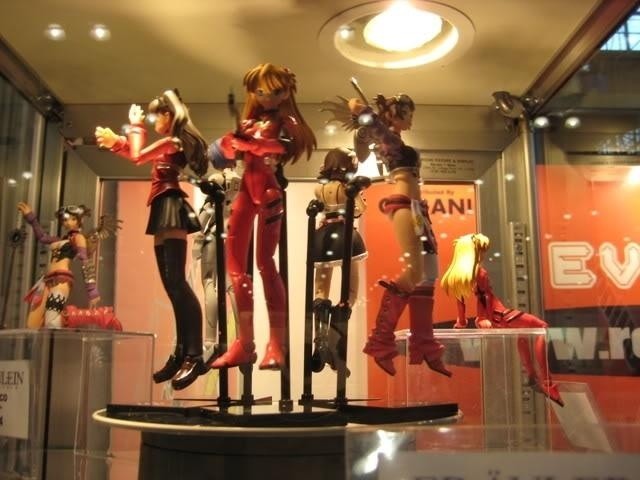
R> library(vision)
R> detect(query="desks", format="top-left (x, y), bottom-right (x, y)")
top-left (90, 408), bottom-right (463, 479)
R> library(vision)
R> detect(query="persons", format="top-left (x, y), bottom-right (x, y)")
top-left (306, 148), bottom-right (372, 376)
top-left (192, 143), bottom-right (243, 376)
top-left (96, 85), bottom-right (212, 390)
top-left (210, 61), bottom-right (317, 370)
top-left (442, 233), bottom-right (567, 405)
top-left (17, 200), bottom-right (123, 329)
top-left (317, 75), bottom-right (453, 377)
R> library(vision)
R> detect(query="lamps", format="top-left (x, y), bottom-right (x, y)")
top-left (315, 0), bottom-right (477, 77)
